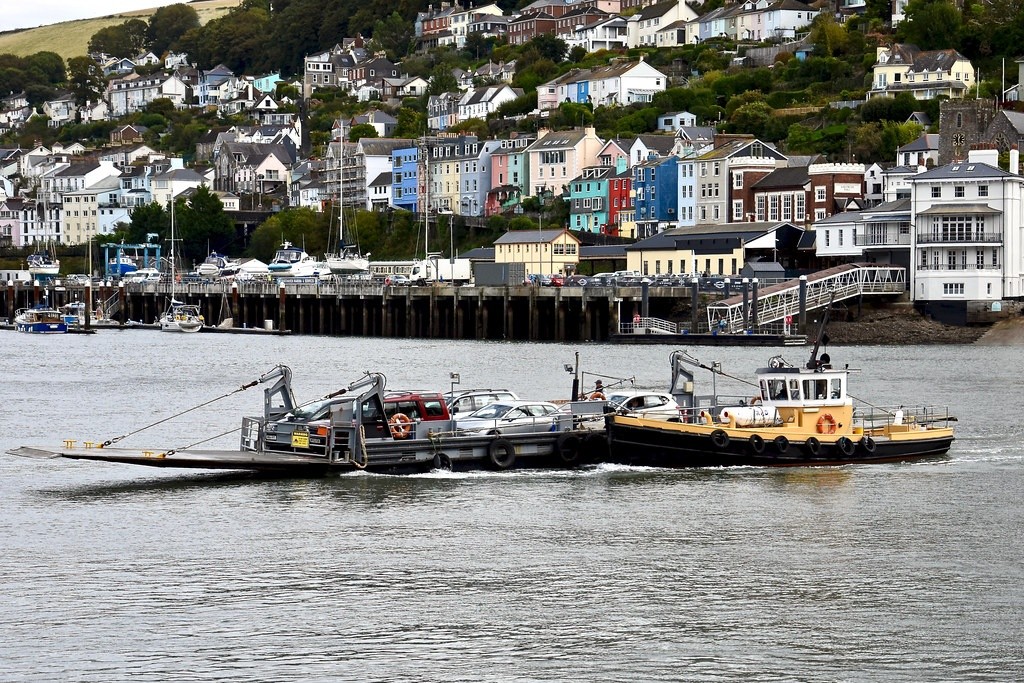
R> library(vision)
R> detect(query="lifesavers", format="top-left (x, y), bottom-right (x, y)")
top-left (817, 413), bottom-right (837, 434)
top-left (390, 412), bottom-right (411, 438)
top-left (385, 278), bottom-right (390, 284)
top-left (750, 396), bottom-right (762, 407)
top-left (837, 437), bottom-right (856, 457)
top-left (554, 433), bottom-right (609, 463)
top-left (635, 315), bottom-right (640, 323)
top-left (589, 392), bottom-right (606, 402)
top-left (774, 436), bottom-right (789, 454)
top-left (749, 435), bottom-right (765, 454)
top-left (176, 315), bottom-right (180, 320)
top-left (710, 429), bottom-right (730, 450)
top-left (806, 437), bottom-right (821, 456)
top-left (579, 279), bottom-right (586, 285)
top-left (432, 453), bottom-right (453, 472)
top-left (487, 438), bottom-right (516, 469)
top-left (859, 437), bottom-right (876, 454)
top-left (786, 316), bottom-right (792, 324)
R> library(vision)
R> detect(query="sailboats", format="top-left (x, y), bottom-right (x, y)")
top-left (160, 186), bottom-right (204, 331)
top-left (65, 207), bottom-right (119, 324)
top-left (322, 120), bottom-right (372, 273)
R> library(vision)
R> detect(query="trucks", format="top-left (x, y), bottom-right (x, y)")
top-left (410, 258), bottom-right (470, 287)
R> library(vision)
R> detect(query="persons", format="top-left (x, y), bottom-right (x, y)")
top-left (717, 315), bottom-right (727, 334)
top-left (594, 380), bottom-right (604, 393)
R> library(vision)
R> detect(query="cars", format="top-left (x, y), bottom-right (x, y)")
top-left (67, 273), bottom-right (120, 285)
top-left (385, 275), bottom-right (412, 287)
top-left (544, 274), bottom-right (564, 286)
top-left (565, 271), bottom-right (739, 287)
top-left (454, 401), bottom-right (566, 436)
top-left (527, 274), bottom-right (551, 286)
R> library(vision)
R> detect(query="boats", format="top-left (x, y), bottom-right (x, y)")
top-left (13, 285), bottom-right (67, 333)
top-left (6, 351), bottom-right (626, 471)
top-left (108, 237), bottom-right (138, 279)
top-left (26, 210), bottom-right (60, 279)
top-left (198, 239), bottom-right (242, 278)
top-left (603, 289), bottom-right (959, 466)
top-left (267, 232), bottom-right (317, 277)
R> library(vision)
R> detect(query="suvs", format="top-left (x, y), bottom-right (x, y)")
top-left (576, 390), bottom-right (684, 429)
top-left (440, 390), bottom-right (520, 420)
top-left (381, 390), bottom-right (452, 439)
